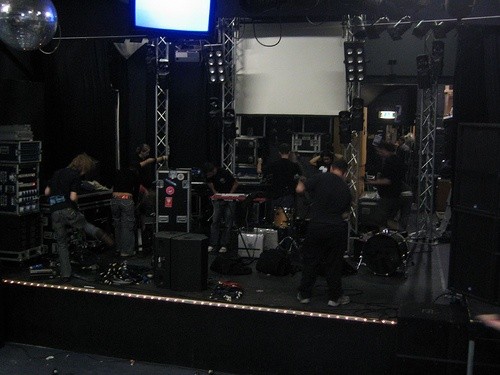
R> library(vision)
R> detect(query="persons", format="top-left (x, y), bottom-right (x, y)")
top-left (365, 139), bottom-right (405, 235)
top-left (44, 152), bottom-right (114, 287)
top-left (122, 144), bottom-right (169, 217)
top-left (101, 149), bottom-right (136, 257)
top-left (295, 157), bottom-right (350, 306)
top-left (201, 160), bottom-right (235, 253)
top-left (371, 128), bottom-right (416, 165)
top-left (256, 142), bottom-right (347, 254)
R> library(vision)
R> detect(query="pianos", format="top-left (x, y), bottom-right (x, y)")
top-left (210, 193), bottom-right (268, 259)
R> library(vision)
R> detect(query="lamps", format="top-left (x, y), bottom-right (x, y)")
top-left (390, 16), bottom-right (414, 41)
top-left (351, 16), bottom-right (367, 38)
top-left (378, 106), bottom-right (398, 119)
top-left (432, 19), bottom-right (458, 37)
top-left (367, 17), bottom-right (392, 40)
top-left (413, 19), bottom-right (434, 38)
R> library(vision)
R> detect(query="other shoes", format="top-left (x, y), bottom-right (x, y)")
top-left (208, 246), bottom-right (213, 252)
top-left (219, 246), bottom-right (227, 252)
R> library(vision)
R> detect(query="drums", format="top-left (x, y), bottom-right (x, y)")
top-left (271, 205), bottom-right (296, 230)
top-left (363, 231), bottom-right (409, 275)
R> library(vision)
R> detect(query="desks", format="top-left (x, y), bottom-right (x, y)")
top-left (210, 197), bottom-right (268, 262)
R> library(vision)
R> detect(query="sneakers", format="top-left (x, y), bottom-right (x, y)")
top-left (296, 291), bottom-right (310, 304)
top-left (328, 295), bottom-right (351, 307)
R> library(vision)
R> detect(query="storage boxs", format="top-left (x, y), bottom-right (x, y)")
top-left (154, 170), bottom-right (190, 234)
top-left (0, 140), bottom-right (42, 163)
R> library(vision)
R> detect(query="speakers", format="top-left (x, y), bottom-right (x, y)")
top-left (452, 121), bottom-right (500, 215)
top-left (445, 207), bottom-right (500, 306)
top-left (152, 231), bottom-right (186, 287)
top-left (171, 233), bottom-right (208, 290)
